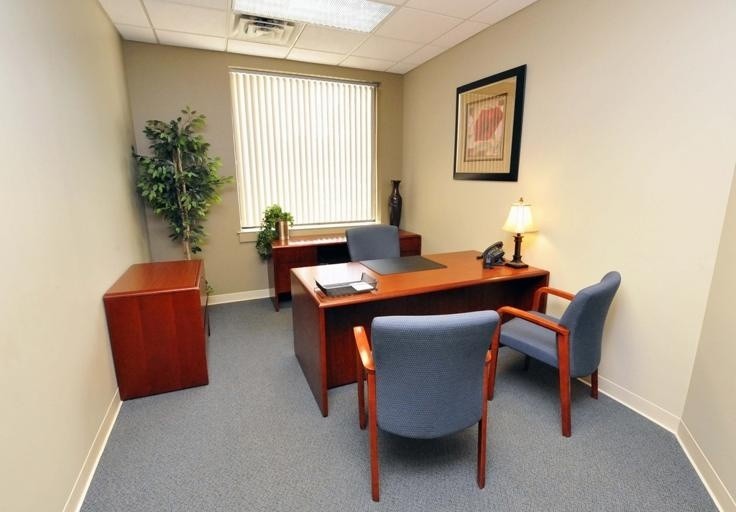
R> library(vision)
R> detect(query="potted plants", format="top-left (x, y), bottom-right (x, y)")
top-left (255, 204), bottom-right (295, 261)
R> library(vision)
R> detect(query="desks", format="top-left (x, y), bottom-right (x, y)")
top-left (289, 250), bottom-right (550, 417)
top-left (267, 229), bottom-right (422, 312)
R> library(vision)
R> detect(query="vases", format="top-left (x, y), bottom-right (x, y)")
top-left (388, 180), bottom-right (402, 227)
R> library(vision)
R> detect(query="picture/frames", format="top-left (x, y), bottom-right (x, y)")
top-left (452, 63), bottom-right (527, 182)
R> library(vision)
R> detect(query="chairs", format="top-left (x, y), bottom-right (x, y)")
top-left (484, 271), bottom-right (622, 435)
top-left (345, 224), bottom-right (401, 262)
top-left (354, 306), bottom-right (501, 500)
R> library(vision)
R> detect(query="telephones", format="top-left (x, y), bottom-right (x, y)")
top-left (483, 240), bottom-right (504, 266)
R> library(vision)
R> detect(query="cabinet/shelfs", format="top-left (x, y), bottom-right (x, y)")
top-left (103, 258), bottom-right (212, 401)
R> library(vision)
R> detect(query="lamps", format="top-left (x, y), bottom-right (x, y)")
top-left (504, 197), bottom-right (539, 269)
